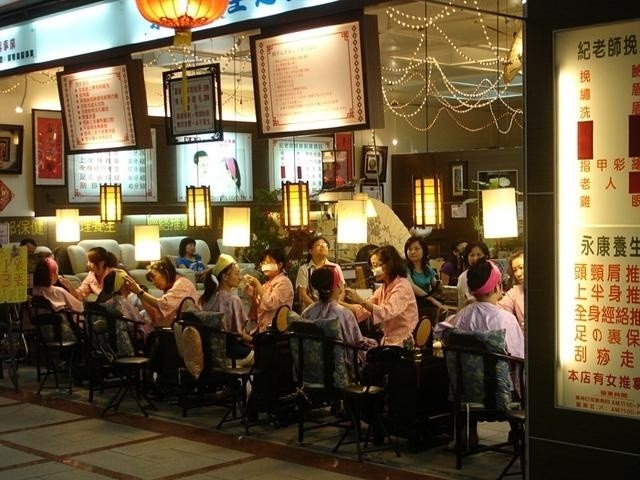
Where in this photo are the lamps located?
[280,136,310,231]
[186,45,211,229]
[337,133,368,243]
[411,0,446,231]
[482,0,519,239]
[135,148,162,262]
[223,35,251,247]
[56,186,80,242]
[98,150,122,224]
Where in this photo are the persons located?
[201,243,295,424]
[99,257,201,392]
[217,158,246,200]
[294,236,445,410]
[174,238,209,283]
[186,150,208,187]
[20,238,37,255]
[440,240,526,443]
[21,247,145,338]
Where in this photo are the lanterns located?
[134,0,229,113]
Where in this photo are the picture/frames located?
[249,17,370,138]
[55,58,138,155]
[162,63,223,145]
[360,182,384,204]
[447,160,468,203]
[32,108,68,188]
[0,124,24,174]
[361,144,388,183]
